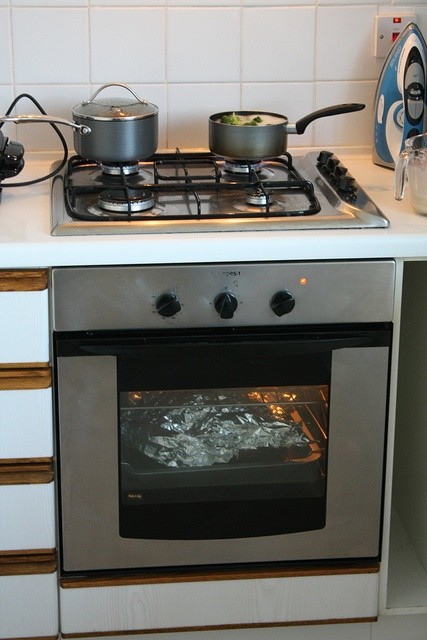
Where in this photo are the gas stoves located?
[49,147,391,237]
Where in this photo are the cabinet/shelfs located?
[0,245,57,639]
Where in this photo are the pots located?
[0,82,160,159]
[208,101,365,161]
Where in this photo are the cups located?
[393,130,427,218]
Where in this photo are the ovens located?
[51,259,397,582]
